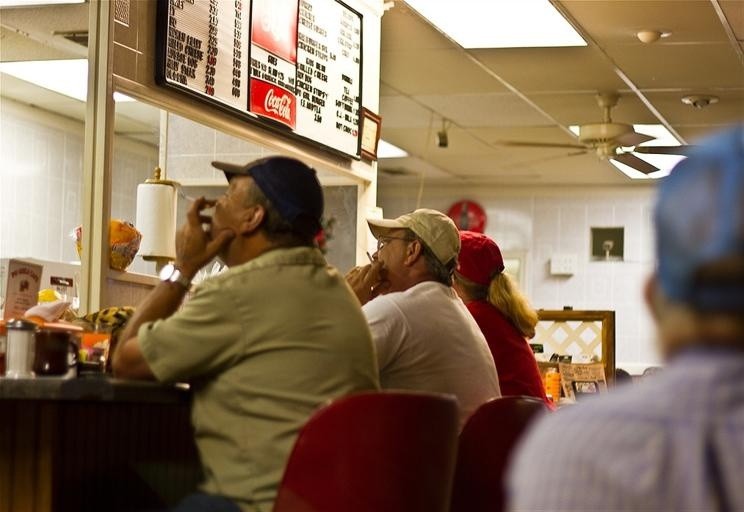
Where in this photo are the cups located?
[33,331,80,378]
[72,321,116,376]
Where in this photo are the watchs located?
[159,263,192,292]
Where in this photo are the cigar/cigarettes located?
[366,251,373,263]
[181,194,212,209]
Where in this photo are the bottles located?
[5,318,37,379]
[541,365,557,400]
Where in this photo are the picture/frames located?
[154,0,364,161]
[501,250,528,293]
[361,107,383,162]
[535,309,616,390]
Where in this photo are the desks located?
[0,374,192,511]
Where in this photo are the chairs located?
[450,394,548,511]
[270,390,462,511]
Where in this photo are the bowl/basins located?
[72,219,143,270]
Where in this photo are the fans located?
[494,91,696,175]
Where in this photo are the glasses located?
[376,234,414,252]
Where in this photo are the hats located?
[649,122,742,318]
[367,207,461,271]
[210,154,325,236]
[456,229,505,286]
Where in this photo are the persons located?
[501,125,743,512]
[111,156,380,512]
[343,208,503,432]
[450,230,554,412]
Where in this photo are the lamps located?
[681,95,719,110]
[137,167,179,263]
